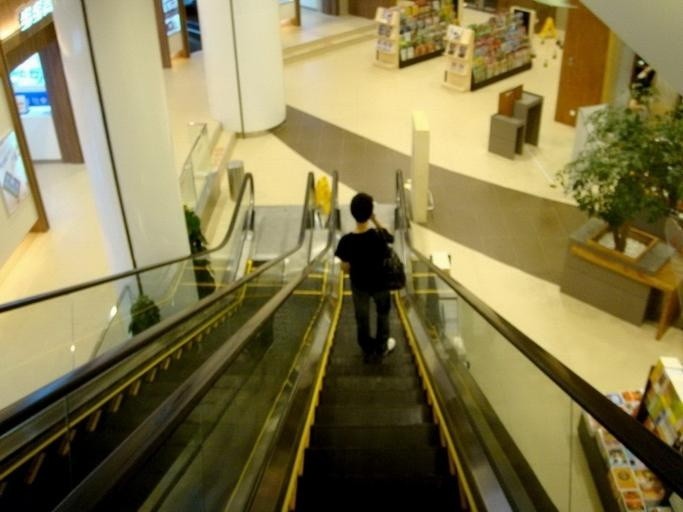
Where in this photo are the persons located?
[334,193,396,357]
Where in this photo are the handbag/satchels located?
[383,248,405,289]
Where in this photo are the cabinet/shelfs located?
[443,12,535,91]
[374,0,456,69]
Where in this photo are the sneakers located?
[383,337,396,357]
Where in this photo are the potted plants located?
[550,81,683,345]
[181,204,218,298]
